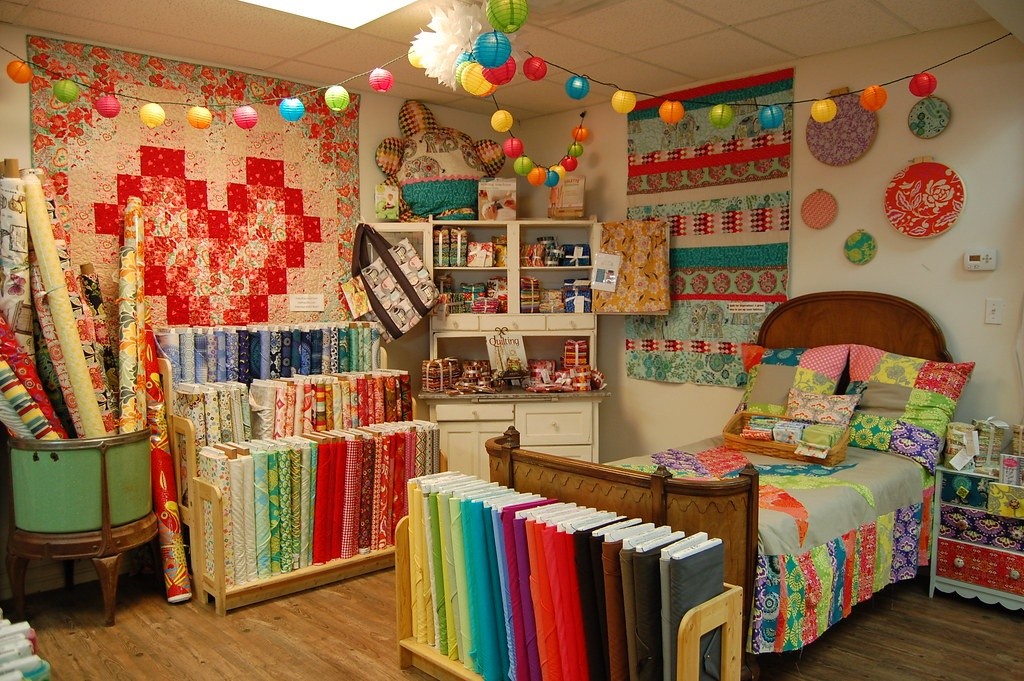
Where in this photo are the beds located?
[485,290,954,680]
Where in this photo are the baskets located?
[723,411,850,466]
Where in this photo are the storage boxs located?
[6,427,153,533]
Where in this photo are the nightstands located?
[929,462,1024,611]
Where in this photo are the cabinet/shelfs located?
[157,346,449,615]
[429,399,598,480]
[429,215,600,331]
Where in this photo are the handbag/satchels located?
[341,223,442,344]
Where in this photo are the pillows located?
[732,343,849,423]
[844,342,975,474]
[785,385,863,424]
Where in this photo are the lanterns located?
[1,0,938,189]
